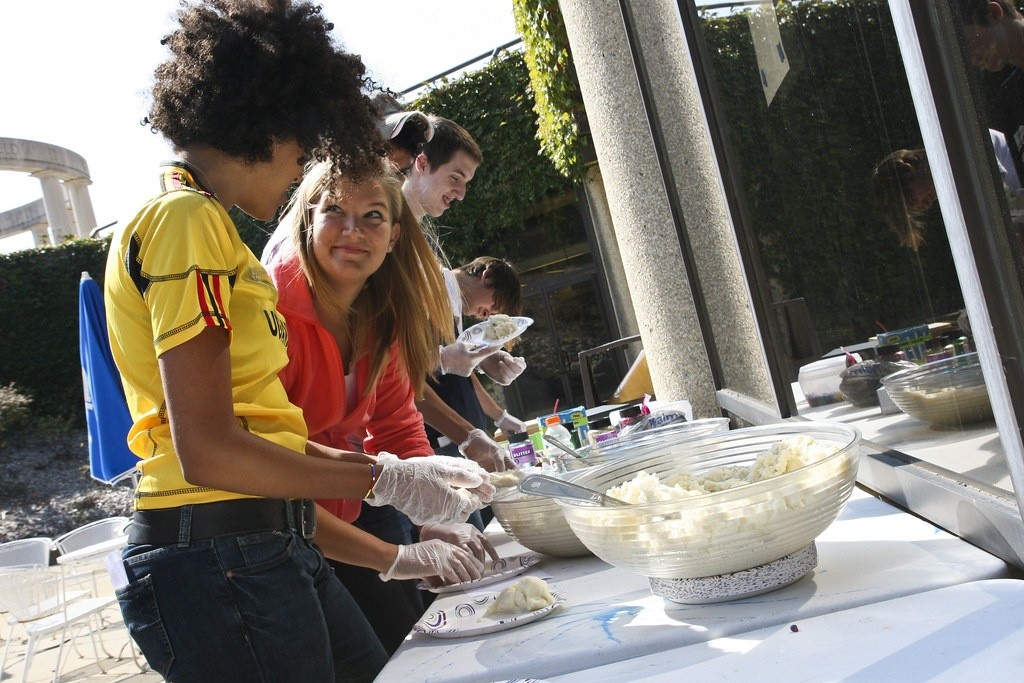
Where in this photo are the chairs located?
[0,515,151,683]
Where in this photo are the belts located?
[127,499,315,544]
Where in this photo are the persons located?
[257,151,500,660]
[451,255,526,438]
[104,0,494,683]
[400,113,519,532]
[874,128,1023,252]
[368,92,502,612]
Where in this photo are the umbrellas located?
[79,271,142,489]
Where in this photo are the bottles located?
[507,432,538,470]
[543,416,576,473]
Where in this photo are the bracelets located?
[363,463,375,500]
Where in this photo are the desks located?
[56,534,130,630]
[790,379,1024,499]
[374,484,1024,683]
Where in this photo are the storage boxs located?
[537,406,591,441]
[610,399,692,422]
[491,425,545,459]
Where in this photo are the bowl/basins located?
[553,421,861,579]
[487,466,599,557]
[879,351,993,425]
[557,417,730,473]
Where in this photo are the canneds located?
[618,405,646,430]
[586,415,620,445]
[507,431,538,468]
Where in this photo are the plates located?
[456,316,534,353]
[416,555,544,593]
[413,590,568,638]
